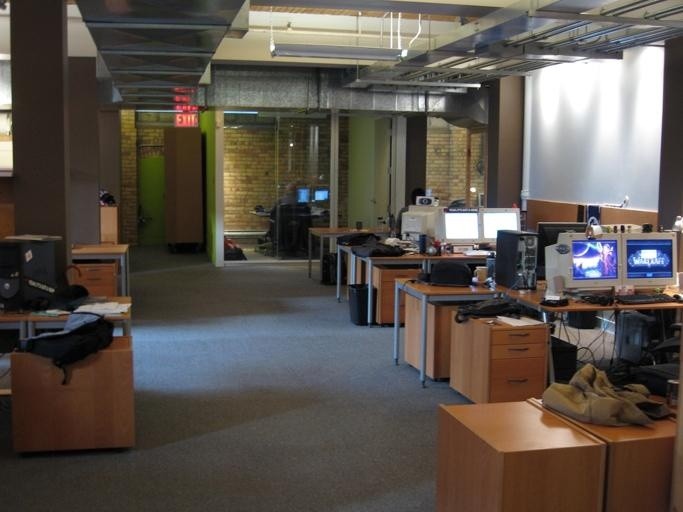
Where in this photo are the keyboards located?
[617,290,671,305]
[462,249,497,257]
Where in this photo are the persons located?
[269,182,312,260]
[394,187,426,241]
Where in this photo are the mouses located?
[669,293,683,304]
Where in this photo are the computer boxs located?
[0,233,66,310]
[494,229,537,291]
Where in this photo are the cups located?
[425,189,432,197]
[474,266,489,282]
[676,272,683,289]
[356,222,363,230]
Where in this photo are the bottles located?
[435,197,439,206]
[377,216,386,233]
[434,240,441,256]
[419,234,426,254]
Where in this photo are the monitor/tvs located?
[543,232,621,297]
[312,184,330,202]
[623,234,677,294]
[479,206,522,244]
[401,204,442,244]
[441,208,481,251]
[536,219,588,277]
[296,184,312,204]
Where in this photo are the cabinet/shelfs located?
[71,258,118,296]
[10,337,137,453]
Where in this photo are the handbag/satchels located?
[336,232,420,258]
[321,253,347,285]
[430,261,472,287]
[455,297,522,323]
[17,312,114,369]
[541,362,674,427]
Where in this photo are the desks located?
[1,295,131,340]
[70,243,131,298]
[437,398,677,511]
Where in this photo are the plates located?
[472,276,493,286]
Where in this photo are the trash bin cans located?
[348,283,377,326]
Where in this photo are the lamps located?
[270,8,425,62]
